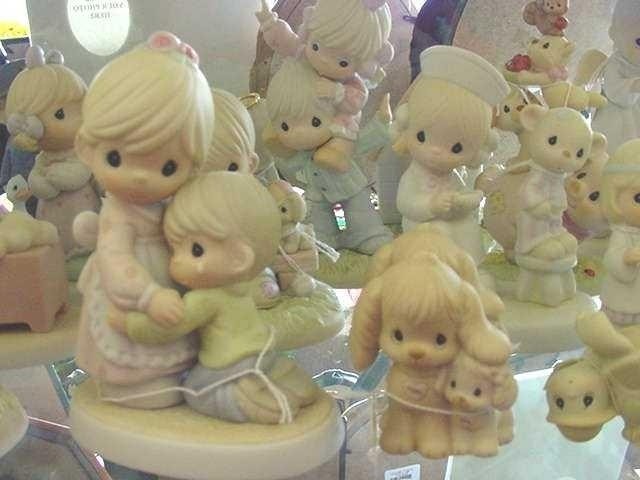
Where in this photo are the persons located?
[0,0,640,459]
[604,139,639,322]
[9,46,98,259]
[251,1,508,318]
[79,27,328,424]
